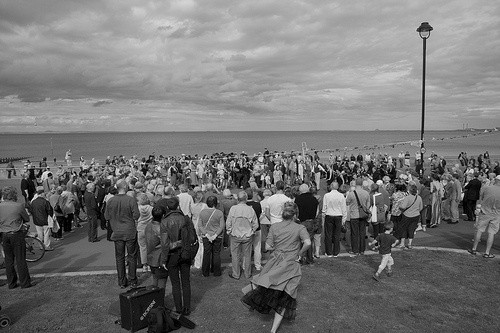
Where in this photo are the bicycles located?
[0,224,45,269]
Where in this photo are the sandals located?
[483,251,495,257]
[468,248,477,254]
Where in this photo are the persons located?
[190,191,207,269]
[322,181,346,258]
[399,185,424,248]
[294,183,320,265]
[468,167,500,258]
[368,220,398,281]
[6,162,16,179]
[240,202,311,333]
[104,179,140,289]
[21,147,500,258]
[226,191,259,279]
[266,180,292,225]
[135,193,153,272]
[0,187,34,290]
[145,197,199,316]
[199,195,225,277]
[346,179,370,255]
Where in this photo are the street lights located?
[416,22,433,164]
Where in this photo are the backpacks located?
[148,305,181,333]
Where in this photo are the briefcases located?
[119,285,165,333]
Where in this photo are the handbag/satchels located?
[195,242,204,269]
[368,194,377,222]
[359,204,372,218]
